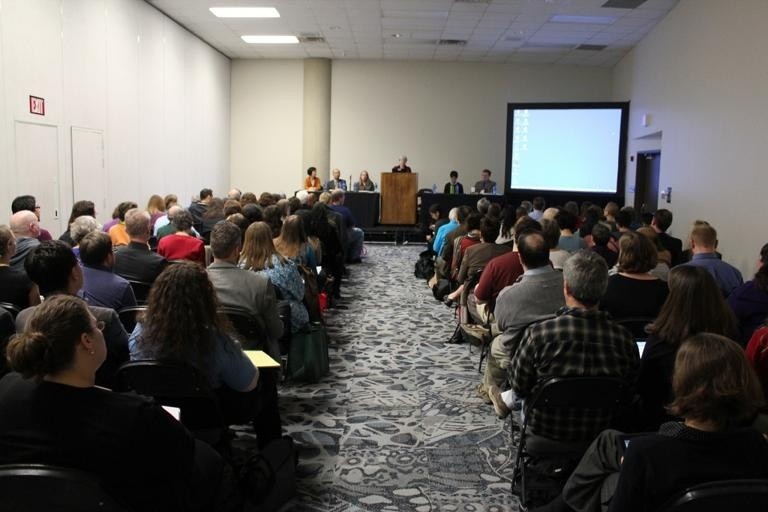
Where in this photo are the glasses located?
[77,322,105,345]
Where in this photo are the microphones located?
[349,176,352,192]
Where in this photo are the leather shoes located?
[325,276,334,289]
[442,294,453,306]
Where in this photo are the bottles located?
[373,182,377,192]
[450,185,454,195]
[341,181,346,189]
[455,184,459,194]
[432,184,437,193]
[492,186,496,194]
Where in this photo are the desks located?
[292,189,382,229]
[420,191,625,231]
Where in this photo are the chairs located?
[449,254,766,512]
[0,204,345,512]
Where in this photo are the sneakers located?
[489,385,512,418]
[476,384,493,405]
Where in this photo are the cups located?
[323,184,327,192]
[471,187,475,192]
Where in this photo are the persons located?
[391,154,412,173]
[443,171,465,193]
[430,197,768,512]
[472,168,500,193]
[0,188,364,512]
[327,169,347,192]
[352,170,374,190]
[303,167,322,191]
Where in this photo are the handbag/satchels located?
[433,279,452,300]
[448,323,491,346]
[282,319,329,384]
[415,249,438,278]
